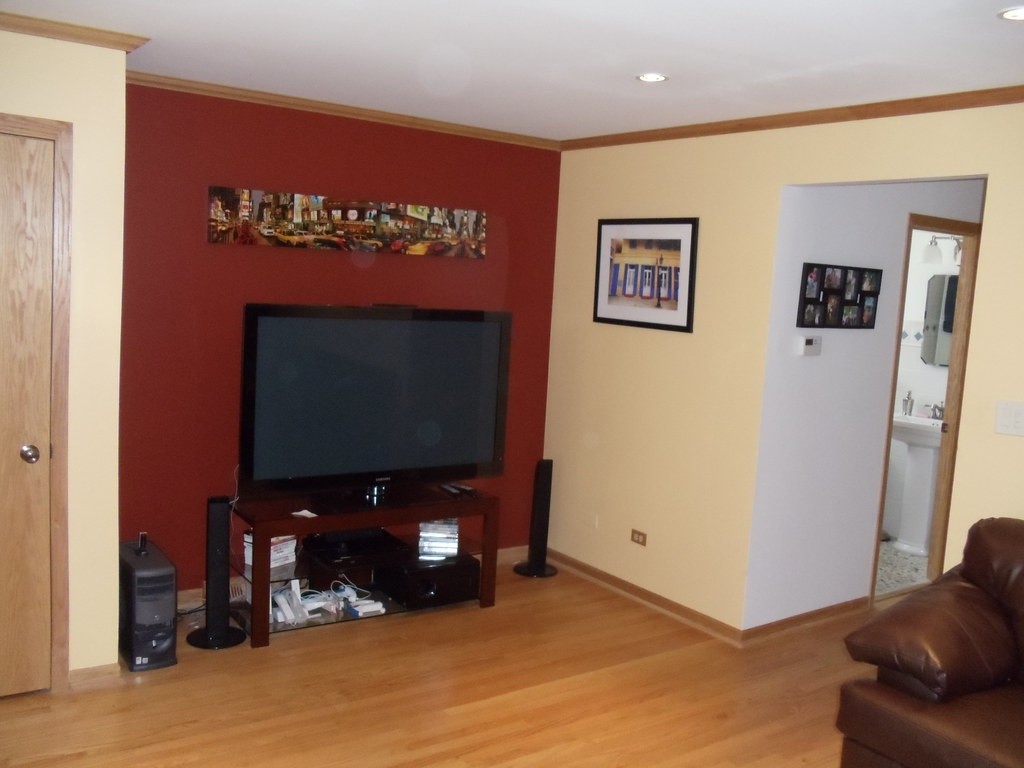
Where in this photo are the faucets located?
[929,403,944,419]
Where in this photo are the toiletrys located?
[900,390,915,415]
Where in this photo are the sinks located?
[891,413,944,448]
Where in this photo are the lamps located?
[920,232,964,267]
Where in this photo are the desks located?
[227,479,503,648]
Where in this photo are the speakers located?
[513,459,557,577]
[186,496,246,649]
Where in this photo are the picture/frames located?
[591,217,698,334]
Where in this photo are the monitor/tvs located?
[237,302,512,493]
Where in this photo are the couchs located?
[835,517,1024,768]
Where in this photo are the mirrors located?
[920,272,959,367]
